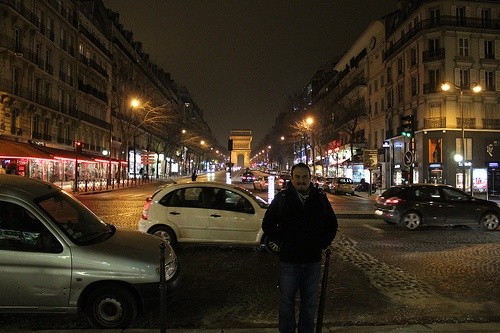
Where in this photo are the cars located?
[374,182,500,231]
[137,181,281,257]
[0,172,184,330]
[232,170,356,196]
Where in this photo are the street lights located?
[304,116,315,166]
[182,129,186,173]
[440,80,482,192]
[128,97,142,186]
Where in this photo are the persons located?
[215,189,228,202]
[261,162,339,333]
[192,170,197,181]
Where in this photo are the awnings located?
[0,140,126,164]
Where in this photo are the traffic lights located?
[401,114,414,138]
